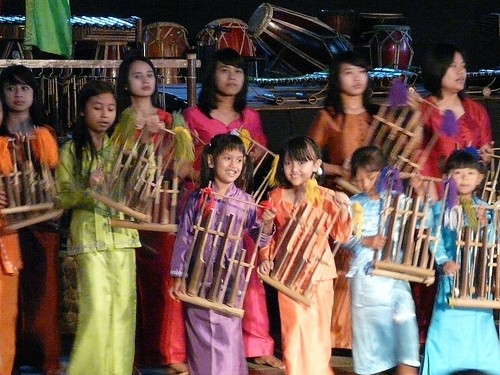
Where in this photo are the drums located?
[359,12,405,61]
[197,18,257,77]
[247,3,357,76]
[318,9,357,43]
[367,24,414,71]
[141,22,190,85]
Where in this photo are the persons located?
[310,51,423,349]
[175,48,285,369]
[169,133,278,374]
[339,145,439,375]
[412,47,494,200]
[107,55,189,374]
[418,146,500,375]
[0,65,59,375]
[257,136,353,374]
[54,80,165,374]
